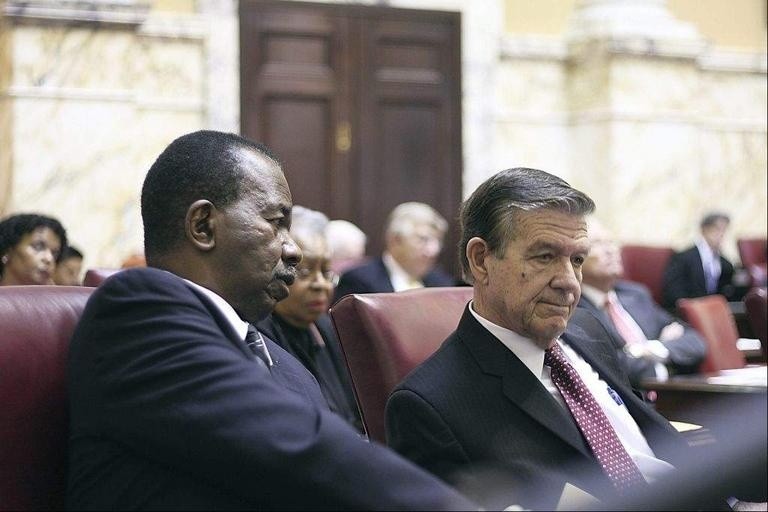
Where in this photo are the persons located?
[330,199,455,299]
[567,208,706,386]
[251,203,365,438]
[61,129,530,512]
[324,217,367,275]
[383,166,766,512]
[660,212,750,309]
[51,246,83,287]
[0,211,66,287]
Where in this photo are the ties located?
[545,344,650,501]
[244,323,270,369]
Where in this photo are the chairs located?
[0,239,768,512]
